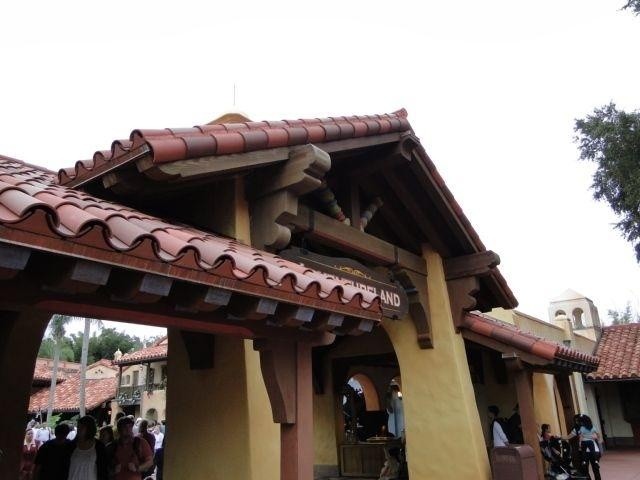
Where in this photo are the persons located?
[378,374,408,480]
[541,413,602,480]
[19,397,165,480]
[487,401,523,447]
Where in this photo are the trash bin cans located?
[493,444,539,480]
